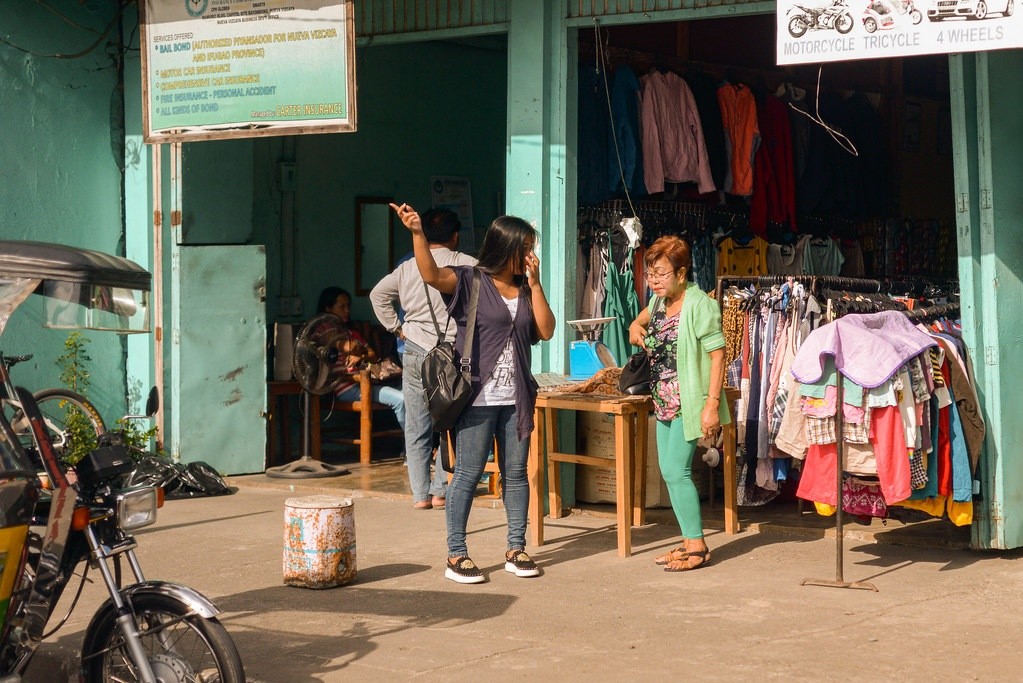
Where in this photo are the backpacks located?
[421,266,481,432]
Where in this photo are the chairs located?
[296,321,404,465]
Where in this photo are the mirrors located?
[355,197,394,298]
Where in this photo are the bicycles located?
[0,350,108,493]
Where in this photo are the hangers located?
[723,67,743,92]
[719,275,962,326]
[577,200,860,257]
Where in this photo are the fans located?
[265,314,352,479]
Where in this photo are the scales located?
[567,317,618,381]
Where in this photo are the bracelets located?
[707,394,722,403]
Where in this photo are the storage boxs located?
[578,411,711,508]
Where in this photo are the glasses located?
[641,267,679,282]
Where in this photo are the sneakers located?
[504,550,539,576]
[444,556,484,583]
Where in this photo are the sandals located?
[664,546,712,571]
[657,548,686,564]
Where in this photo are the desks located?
[526,392,654,557]
[265,382,301,467]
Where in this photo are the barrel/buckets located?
[283,495,357,588]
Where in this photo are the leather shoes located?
[416,498,434,511]
[433,498,445,510]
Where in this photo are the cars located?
[926,0,1016,22]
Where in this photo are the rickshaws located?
[0,236,250,683]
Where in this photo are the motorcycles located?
[860,0,923,34]
[785,0,855,39]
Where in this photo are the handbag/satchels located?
[620,353,650,394]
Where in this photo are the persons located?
[629,234,732,572]
[388,202,556,585]
[369,208,479,509]
[308,286,405,432]
